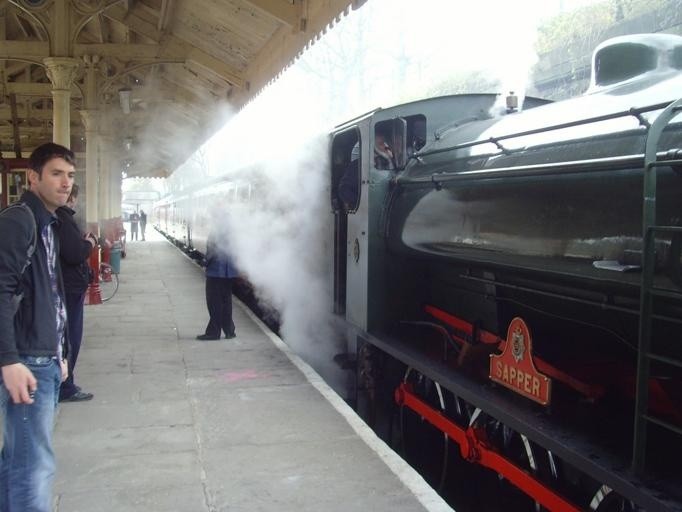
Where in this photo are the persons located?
[338,133,395,211]
[54,181,99,402]
[139,209,148,242]
[197,196,246,341]
[129,210,138,238]
[2,139,73,511]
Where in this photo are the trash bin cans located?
[110,241,122,274]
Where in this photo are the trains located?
[147,29,682,512]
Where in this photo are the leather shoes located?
[224,331,237,339]
[197,332,219,341]
[61,390,93,402]
